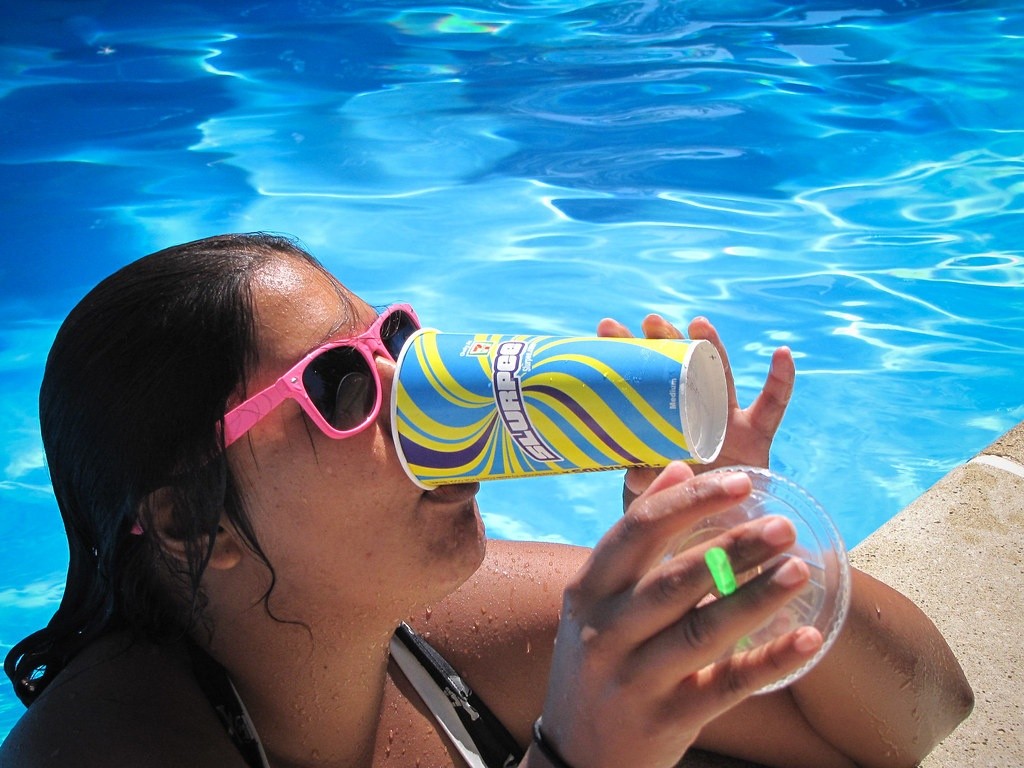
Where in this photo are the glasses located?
[128,302,423,536]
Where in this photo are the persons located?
[0,233,975,768]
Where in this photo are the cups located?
[391,327,728,491]
